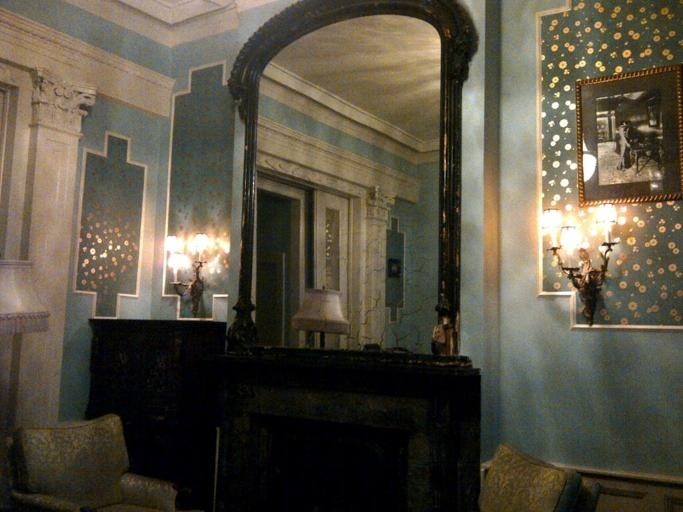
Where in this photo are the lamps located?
[164,232,230,317]
[289,285,352,351]
[0,258,50,431]
[540,202,620,326]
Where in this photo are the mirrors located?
[226,1,479,357]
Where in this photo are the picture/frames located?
[572,63,682,208]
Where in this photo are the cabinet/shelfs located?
[83,319,226,509]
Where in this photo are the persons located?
[615,119,635,173]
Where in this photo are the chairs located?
[0,414,179,511]
[476,439,601,511]
